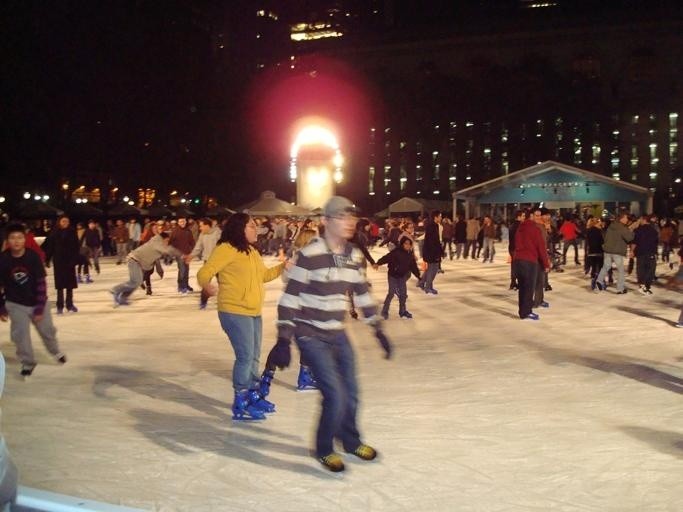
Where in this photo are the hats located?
[323,198,359,220]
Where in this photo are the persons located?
[1,223,71,383]
[354,211,505,320]
[198,213,287,420]
[266,197,389,470]
[0,216,324,314]
[509,206,682,319]
[260,238,320,395]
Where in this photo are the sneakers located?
[320,453,344,473]
[415,282,438,294]
[55,352,66,364]
[66,305,77,312]
[541,302,549,307]
[520,312,538,320]
[400,311,412,318]
[353,442,376,460]
[20,364,35,376]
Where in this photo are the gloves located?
[266,338,292,370]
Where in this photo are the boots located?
[297,365,318,386]
[232,369,274,416]
[77,276,94,283]
